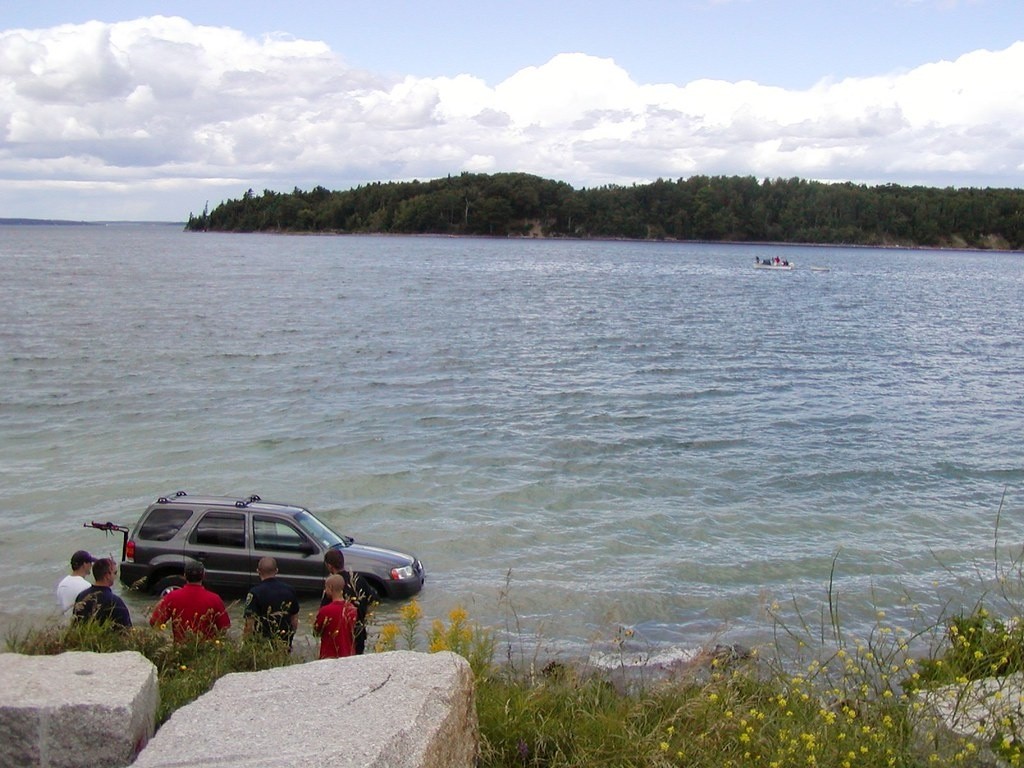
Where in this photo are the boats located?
[752,261,794,271]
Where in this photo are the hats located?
[71,550,98,563]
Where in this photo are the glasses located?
[105,571,118,574]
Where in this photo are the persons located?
[320,549,381,655]
[72,558,133,628]
[753,255,790,267]
[312,575,359,660]
[149,561,233,648]
[59,550,97,619]
[244,557,299,654]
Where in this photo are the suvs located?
[119,489,426,607]
